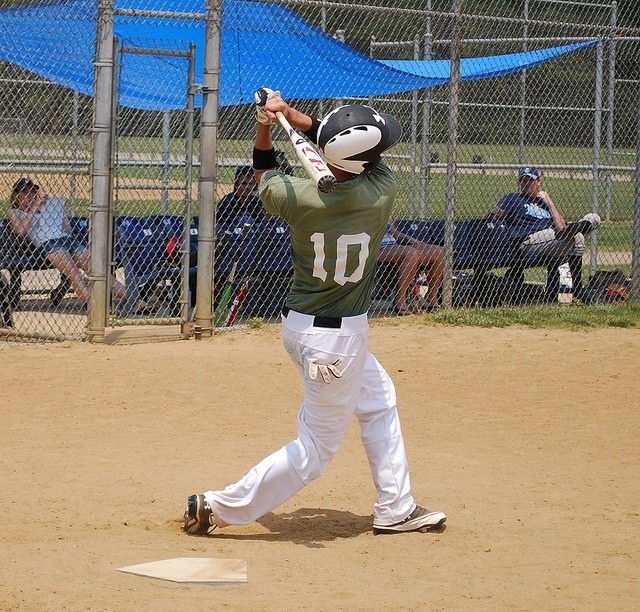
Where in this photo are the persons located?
[486,167,602,306]
[182,84,447,535]
[6,177,159,316]
[217,165,267,217]
[376,214,446,316]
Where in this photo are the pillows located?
[317,105,402,175]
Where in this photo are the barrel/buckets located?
[421,305,442,312]
[393,300,412,316]
[132,305,159,315]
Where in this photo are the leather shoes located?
[254,87,273,127]
[309,356,342,384]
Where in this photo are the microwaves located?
[214,262,238,327]
[223,277,249,328]
[274,110,339,194]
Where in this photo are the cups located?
[235,181,257,186]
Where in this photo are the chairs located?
[572,293,595,305]
[373,505,446,532]
[554,222,585,241]
[0,213,563,314]
[184,494,217,536]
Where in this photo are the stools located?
[281,306,341,329]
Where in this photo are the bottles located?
[12,178,39,192]
[519,167,539,179]
